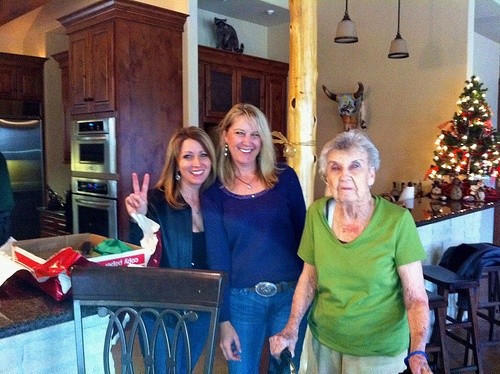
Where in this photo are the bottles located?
[391,181,424,202]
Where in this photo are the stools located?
[423,264,500,374]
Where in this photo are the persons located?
[0,151,15,238]
[200,102,307,372]
[125,124,217,374]
[268,129,432,374]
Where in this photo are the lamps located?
[334,0,358,44]
[387,0,410,60]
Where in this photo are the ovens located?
[69,119,118,240]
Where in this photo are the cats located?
[214,16,245,54]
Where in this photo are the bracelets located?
[404,350,430,366]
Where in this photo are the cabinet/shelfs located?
[0,0,191,239]
[197,45,290,163]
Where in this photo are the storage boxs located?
[8,231,147,301]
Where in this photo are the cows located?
[322,80,368,133]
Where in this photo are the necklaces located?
[188,194,200,215]
[235,175,257,188]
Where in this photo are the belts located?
[244,279,299,298]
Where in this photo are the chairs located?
[68,265,226,374]
